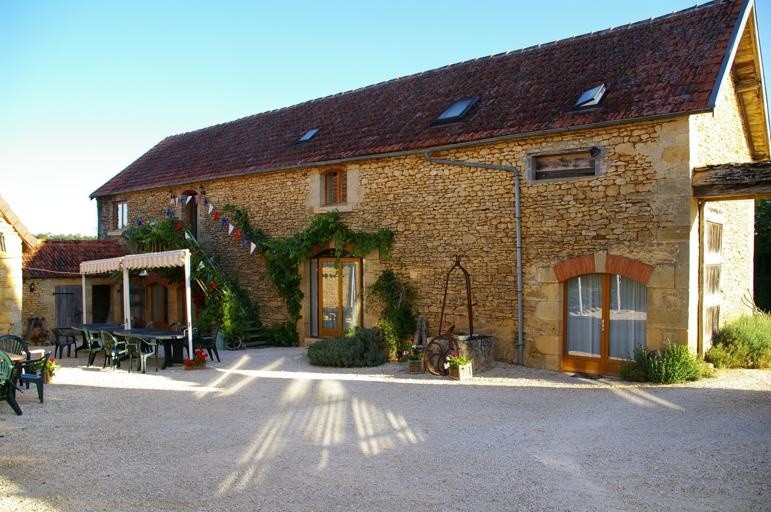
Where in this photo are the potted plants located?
[407,352,426,374]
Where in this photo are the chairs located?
[0,334,53,416]
[51,320,221,373]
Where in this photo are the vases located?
[447,363,473,380]
[43,368,50,383]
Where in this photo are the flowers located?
[31,356,57,373]
[443,352,469,370]
[29,329,49,346]
[183,347,208,368]
